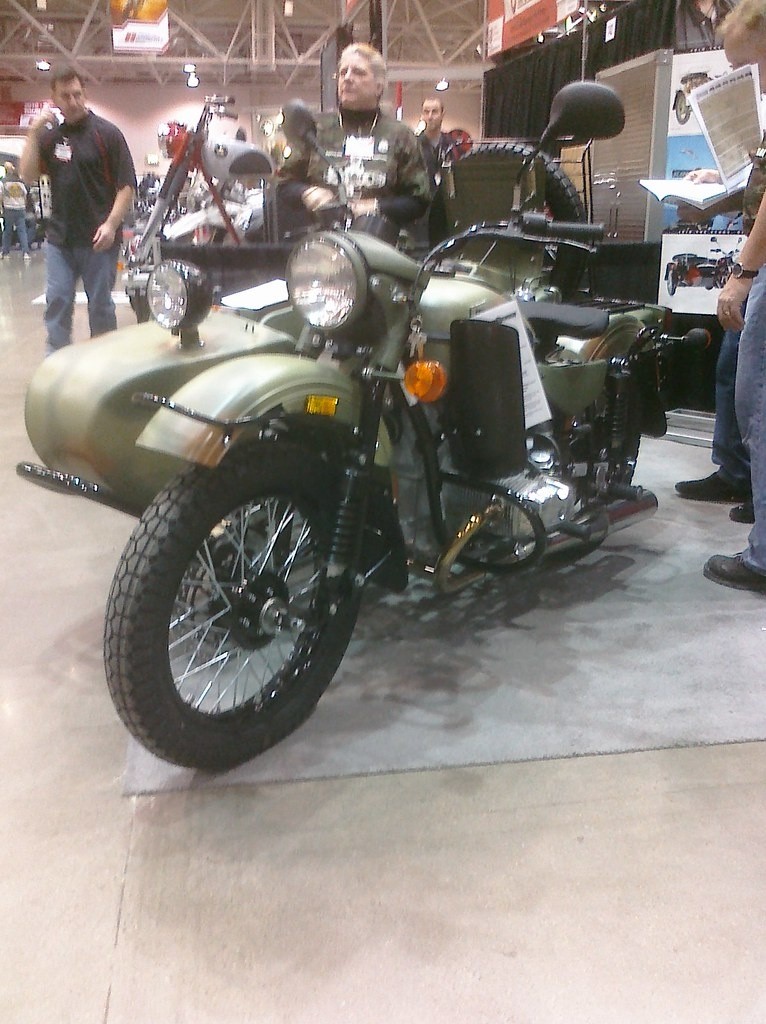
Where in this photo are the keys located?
[408,326,427,358]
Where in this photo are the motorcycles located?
[119,95,444,321]
[671,71,728,125]
[15,76,716,775]
[664,236,744,296]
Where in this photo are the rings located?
[723,311,730,315]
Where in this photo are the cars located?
[0,149,46,253]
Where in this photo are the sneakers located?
[702,554,765,592]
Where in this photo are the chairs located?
[520,300,610,354]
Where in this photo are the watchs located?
[732,263,759,278]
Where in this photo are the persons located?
[675,0,766,591]
[417,98,460,189]
[17,65,135,358]
[277,42,438,220]
[0,162,30,259]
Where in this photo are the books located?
[640,179,747,210]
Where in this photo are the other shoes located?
[728,504,755,525]
[23,253,31,260]
[2,253,10,259]
[674,472,751,503]
